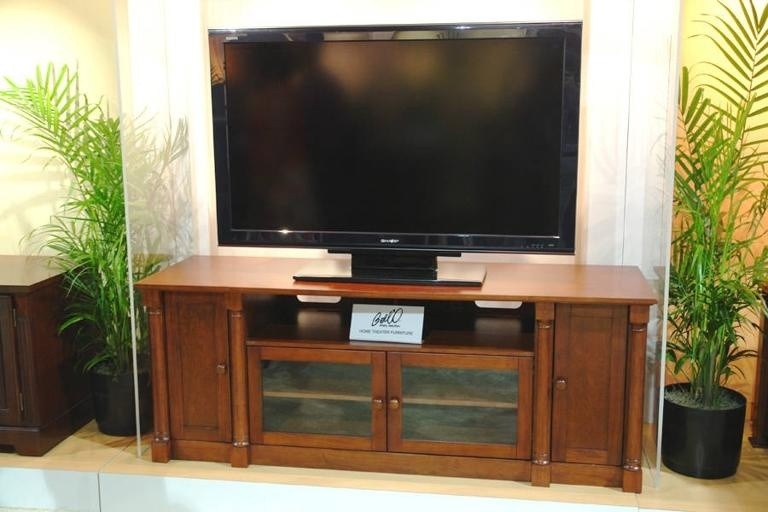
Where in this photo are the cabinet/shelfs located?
[0,254,101,458]
[134,255,658,494]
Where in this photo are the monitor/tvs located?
[208,21,582,287]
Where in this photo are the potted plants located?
[662,0,768,480]
[0,62,193,438]
